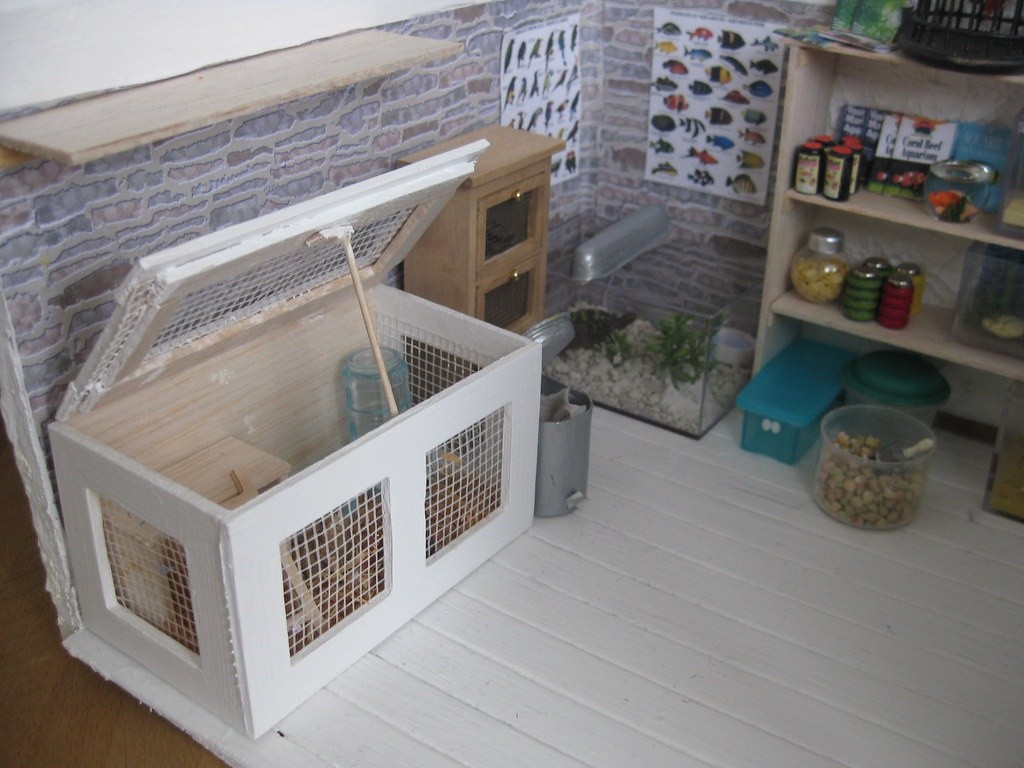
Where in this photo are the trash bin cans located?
[522,310,594,519]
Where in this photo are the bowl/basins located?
[811,403,937,531]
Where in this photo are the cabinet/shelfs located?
[749,27,1024,519]
[394,124,569,415]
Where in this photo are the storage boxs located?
[539,170,770,440]
[46,139,544,739]
[736,340,854,467]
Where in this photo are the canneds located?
[795,136,862,201]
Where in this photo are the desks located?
[103,439,292,630]
